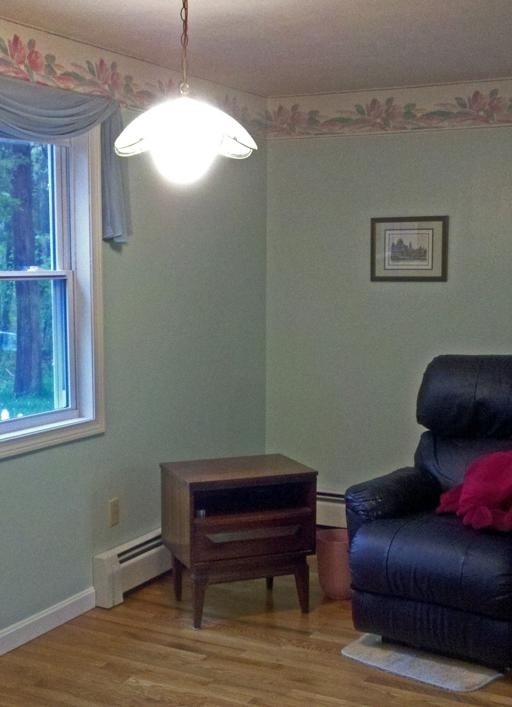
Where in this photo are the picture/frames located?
[369,215,451,284]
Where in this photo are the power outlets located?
[108,497,120,526]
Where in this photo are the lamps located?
[113,1,259,188]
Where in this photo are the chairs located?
[344,354,512,677]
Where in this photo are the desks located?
[160,453,318,628]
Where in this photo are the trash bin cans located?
[317,529,349,598]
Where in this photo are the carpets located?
[342,634,504,695]
